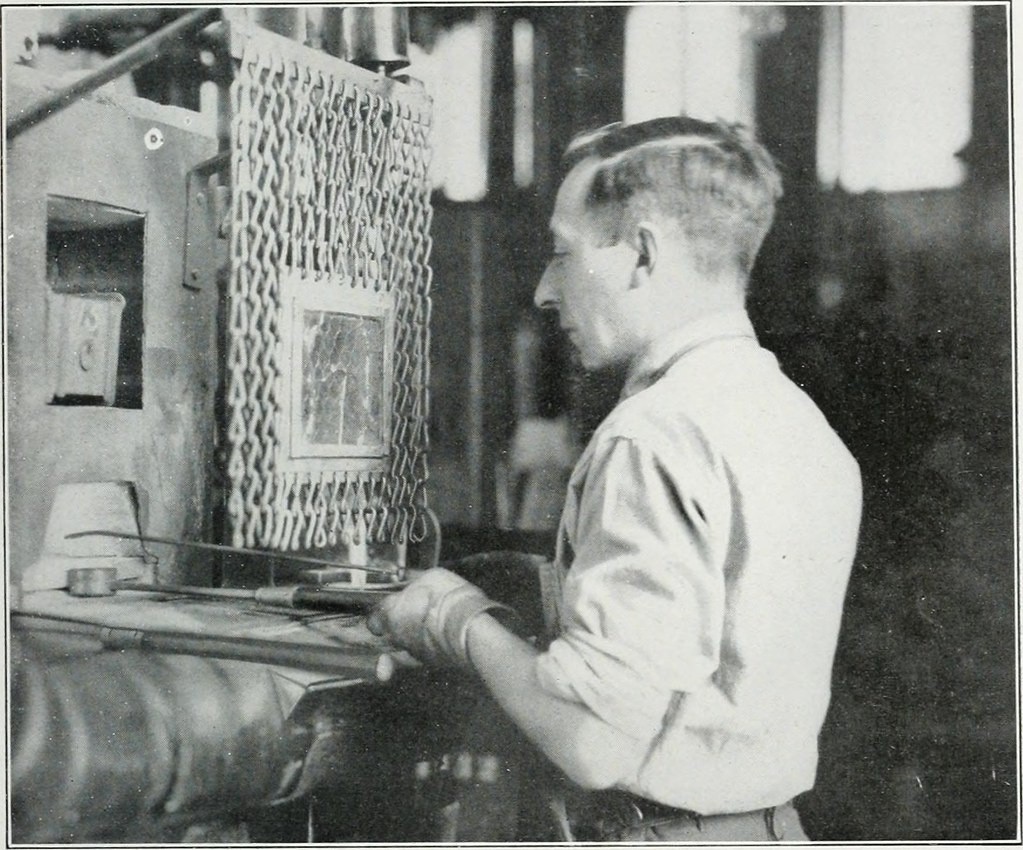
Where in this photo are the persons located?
[366,117,864,842]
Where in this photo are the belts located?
[597,801,690,829]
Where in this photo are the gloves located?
[368,564,528,679]
